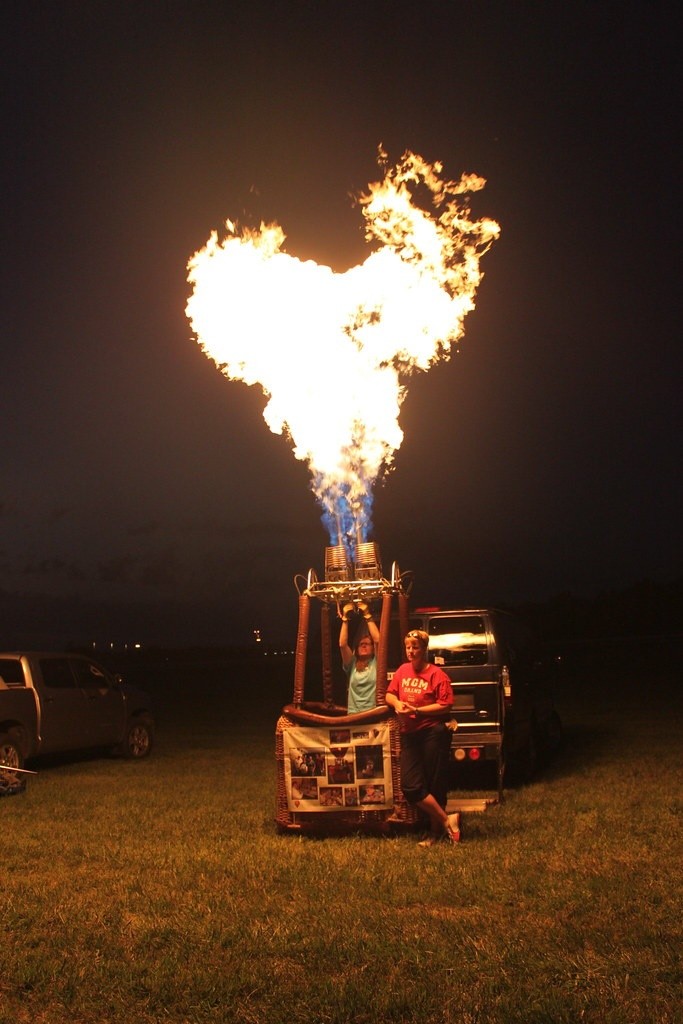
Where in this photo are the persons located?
[384,628,462,850]
[341,601,382,718]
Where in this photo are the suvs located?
[354,610,562,785]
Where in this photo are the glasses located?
[405,633,426,645]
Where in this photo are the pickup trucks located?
[0,651,155,774]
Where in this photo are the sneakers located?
[418,831,441,848]
[445,812,461,846]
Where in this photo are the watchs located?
[414,707,419,717]
